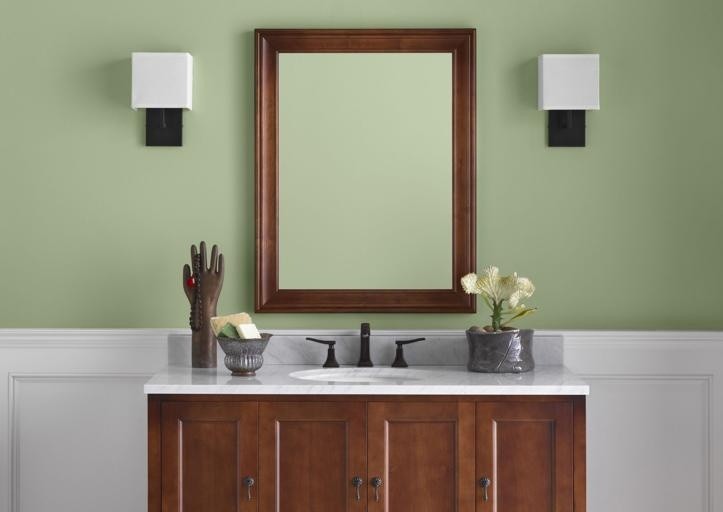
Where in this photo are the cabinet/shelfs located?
[146,395,260,511]
[477,398,574,511]
[259,395,475,512]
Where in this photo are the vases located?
[466,324,536,373]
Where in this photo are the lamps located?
[130,52,194,148]
[537,53,601,147]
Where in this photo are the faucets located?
[357,323,374,367]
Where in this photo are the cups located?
[215,332,271,377]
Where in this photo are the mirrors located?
[253,26,477,314]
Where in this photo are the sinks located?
[288,367,427,383]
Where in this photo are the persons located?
[182,242,224,368]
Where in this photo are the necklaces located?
[186,254,203,332]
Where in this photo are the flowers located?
[461,268,539,331]
